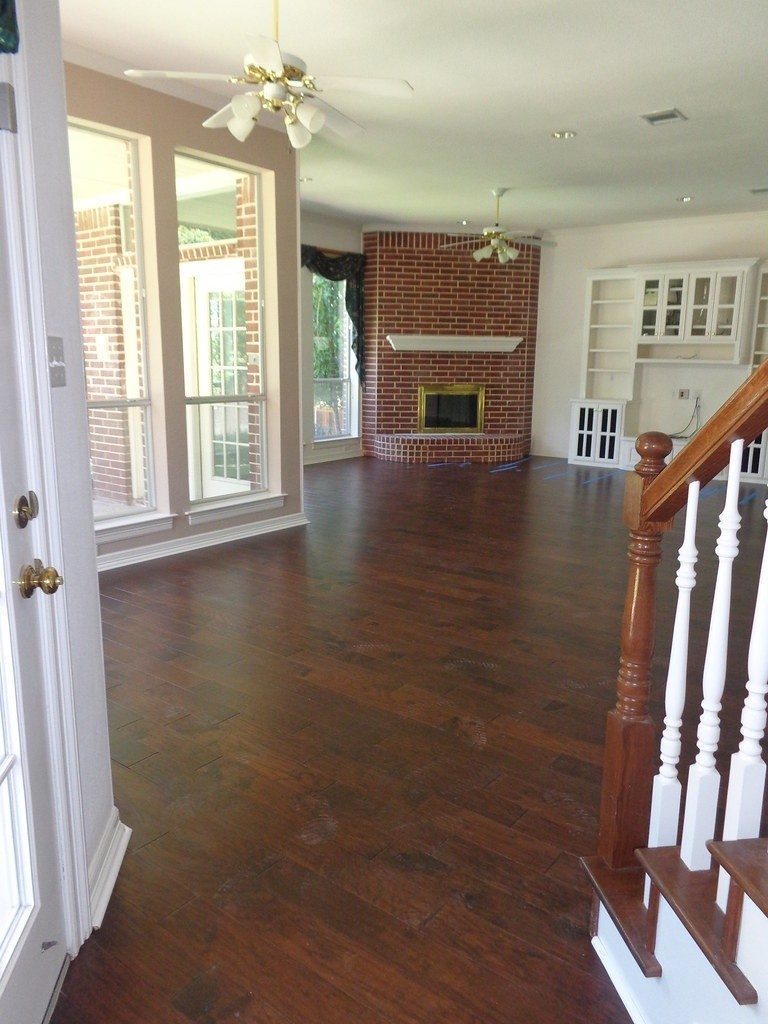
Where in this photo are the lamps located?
[202,51,327,150]
[473,227,520,263]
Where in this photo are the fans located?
[123,0,414,139]
[440,188,557,248]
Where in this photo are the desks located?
[313,404,344,434]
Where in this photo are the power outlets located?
[679,389,689,399]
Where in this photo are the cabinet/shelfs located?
[568,256,768,484]
